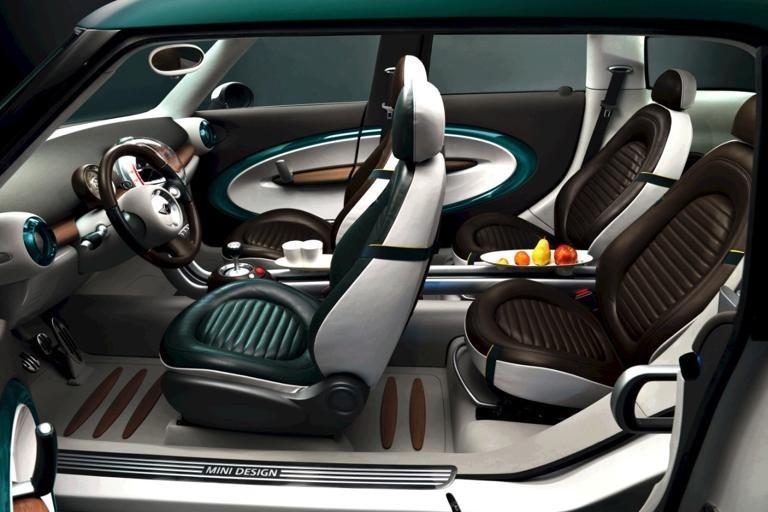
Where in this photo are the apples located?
[554,246,577,265]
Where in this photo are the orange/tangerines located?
[515,252,530,266]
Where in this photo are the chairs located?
[457,67,697,265]
[469,93,755,405]
[153,77,455,434]
[221,56,432,261]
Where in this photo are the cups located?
[282,239,300,264]
[300,239,323,265]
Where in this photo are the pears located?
[532,236,551,266]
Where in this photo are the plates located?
[478,248,592,272]
